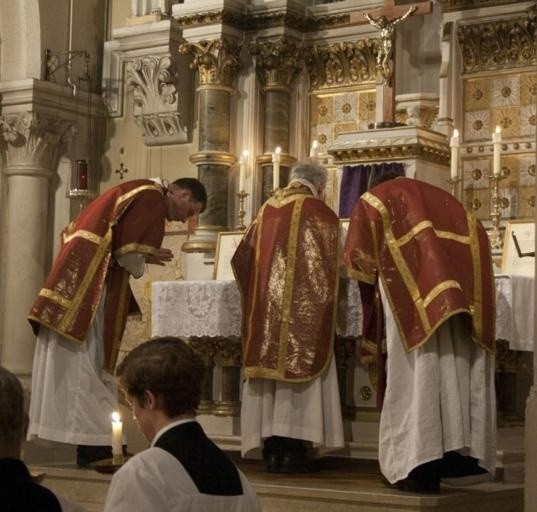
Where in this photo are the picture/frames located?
[211,231,248,282]
[339,217,352,254]
[499,219,537,274]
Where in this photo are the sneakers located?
[76,446,113,466]
[266,454,321,473]
[376,464,442,495]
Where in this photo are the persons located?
[101,333,262,512]
[340,174,502,495]
[229,161,364,476]
[0,363,69,512]
[24,177,207,468]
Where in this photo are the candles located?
[238,140,321,195]
[110,409,123,459]
[449,125,502,180]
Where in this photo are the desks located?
[143,273,533,419]
